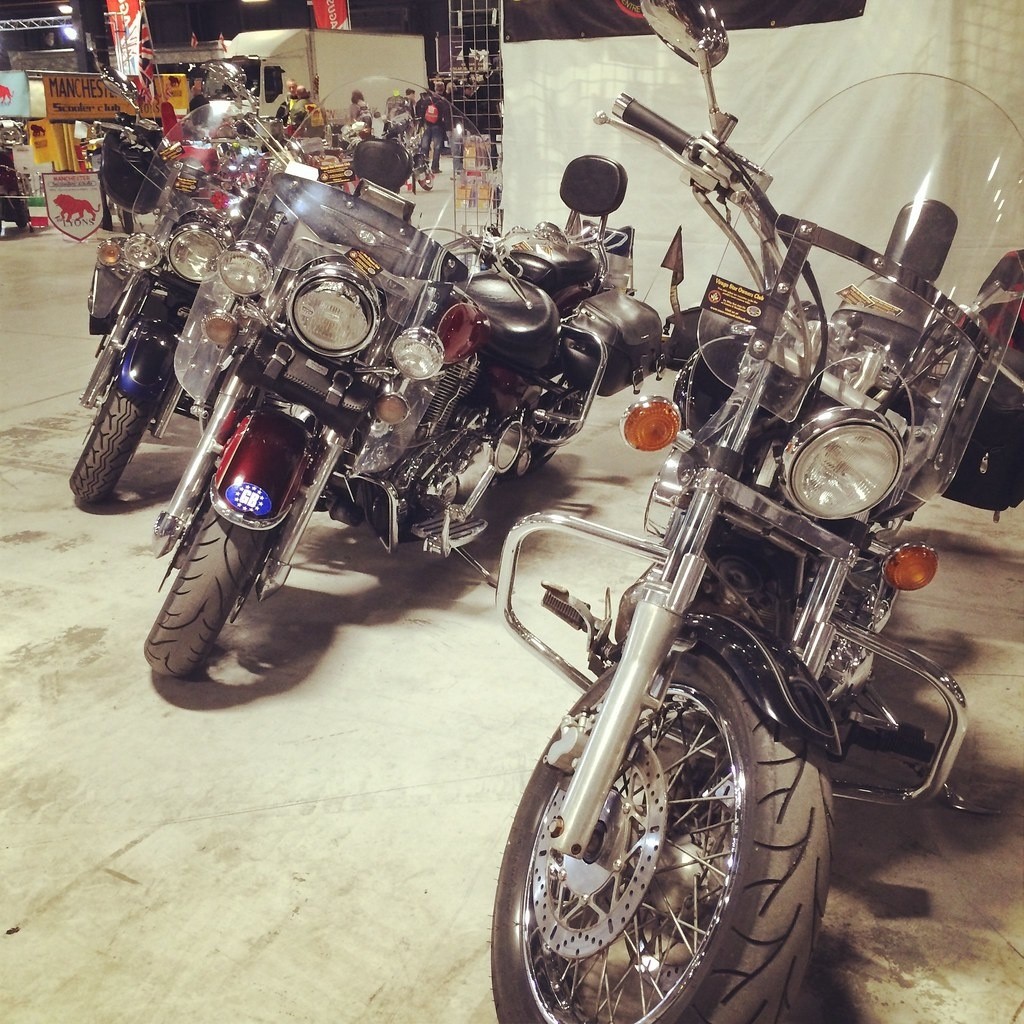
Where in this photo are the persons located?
[350,56,503,174]
[276,78,326,138]
[188,86,209,127]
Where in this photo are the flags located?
[137,1,156,104]
[219,33,227,51]
[190,32,198,49]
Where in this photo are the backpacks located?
[425,97,441,123]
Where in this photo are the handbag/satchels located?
[938,249,1024,510]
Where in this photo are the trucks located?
[0,55,291,236]
[219,29,430,150]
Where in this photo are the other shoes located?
[432,169,441,173]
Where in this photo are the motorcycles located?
[487,0,1024,1024]
[65,58,664,681]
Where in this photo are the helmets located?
[99,132,166,215]
